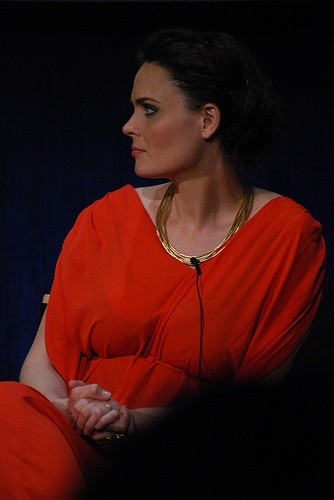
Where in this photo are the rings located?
[107,434,123,440]
[105,404,113,410]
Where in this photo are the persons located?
[0,18,326,500]
[0,352,334,500]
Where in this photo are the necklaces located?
[155,182,254,266]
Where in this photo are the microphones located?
[191,257,201,274]
